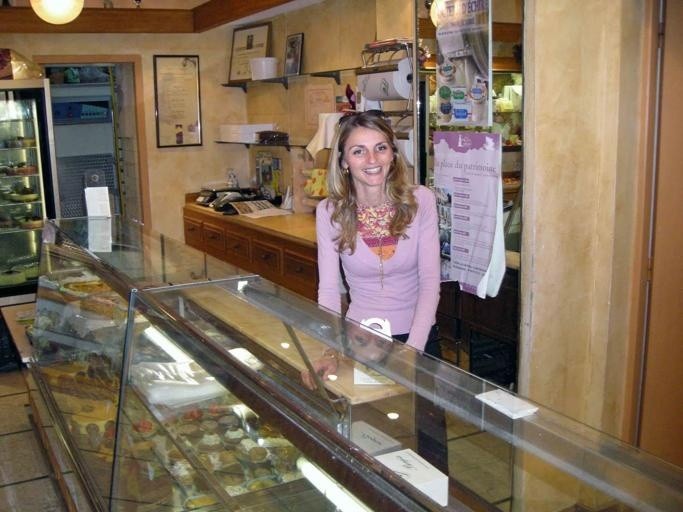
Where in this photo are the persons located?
[300,110,450,475]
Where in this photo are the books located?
[229,199,293,220]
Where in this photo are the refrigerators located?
[0,78,63,307]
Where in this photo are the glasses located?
[338,110,385,127]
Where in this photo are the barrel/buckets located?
[249,57,280,81]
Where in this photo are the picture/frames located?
[151,54,202,148]
[228,21,303,83]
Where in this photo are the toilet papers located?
[356,56,414,101]
[306,112,342,160]
[395,126,414,168]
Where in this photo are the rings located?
[324,350,338,361]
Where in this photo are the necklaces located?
[352,196,395,289]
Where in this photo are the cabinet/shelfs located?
[182,193,317,301]
[212,77,291,152]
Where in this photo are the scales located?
[196,188,256,206]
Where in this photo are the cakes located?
[41,371,296,512]
